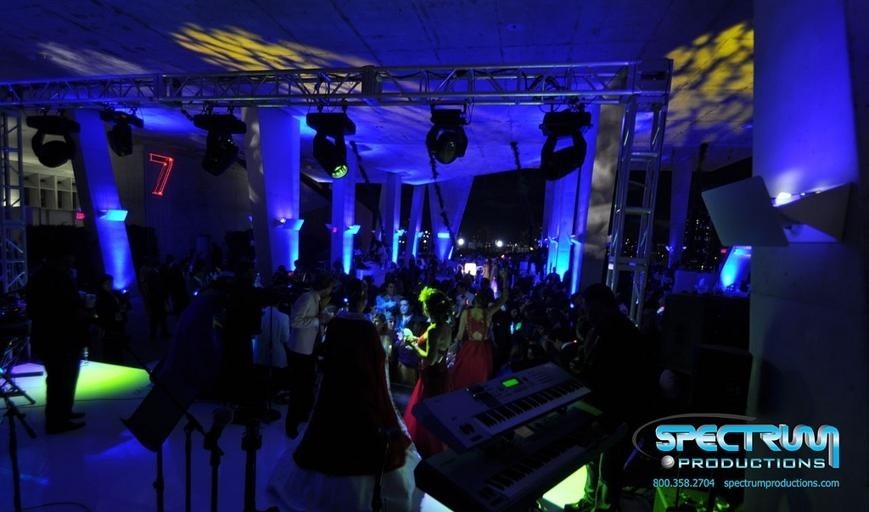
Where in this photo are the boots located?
[371,486,381,512]
[203,407,232,456]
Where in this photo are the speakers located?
[681,346,753,425]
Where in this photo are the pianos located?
[414,401,610,512]
[412,362,591,454]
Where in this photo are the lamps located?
[537,92,592,180]
[99,108,145,158]
[191,113,248,177]
[26,114,80,168]
[426,100,470,164]
[306,111,356,179]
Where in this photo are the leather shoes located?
[285,414,298,438]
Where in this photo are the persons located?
[31,222,698,512]
